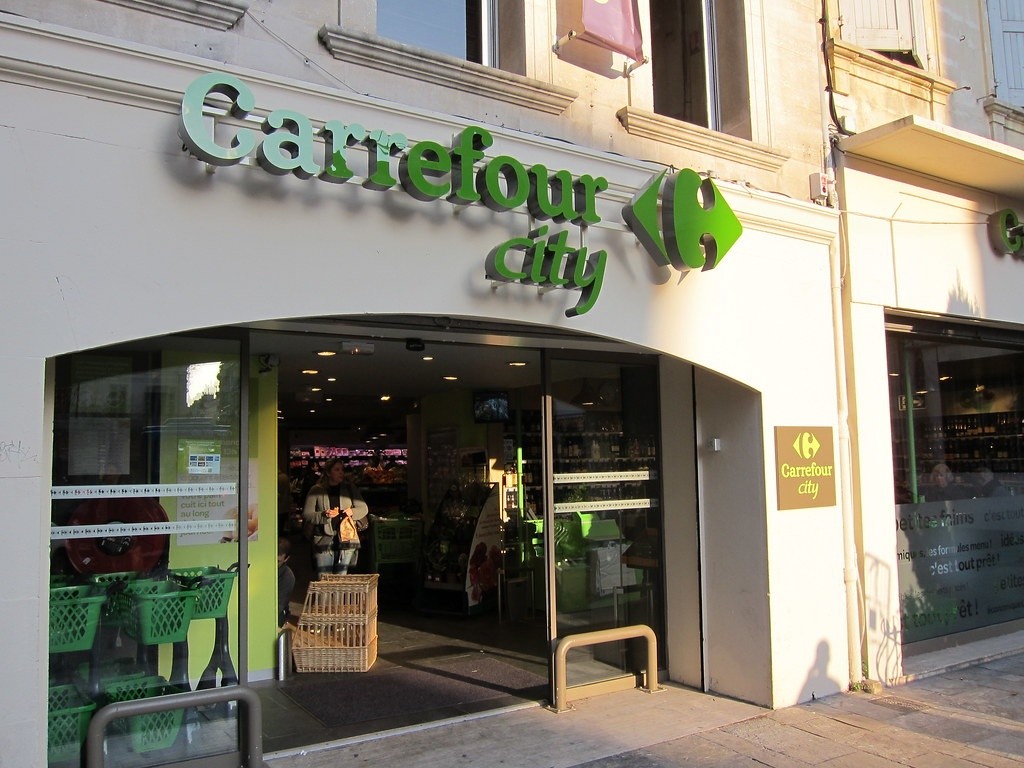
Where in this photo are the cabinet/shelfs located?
[290,454,411,493]
[893,411,1022,505]
[520,429,660,519]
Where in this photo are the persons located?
[278,468,292,538]
[301,458,368,604]
[924,463,967,502]
[277,537,296,616]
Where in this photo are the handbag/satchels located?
[356,516,369,532]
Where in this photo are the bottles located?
[915,414,1024,481]
[524,420,661,516]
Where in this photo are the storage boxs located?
[47,566,238,765]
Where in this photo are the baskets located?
[49,566,236,653]
[291,573,380,673]
[49,663,187,764]
